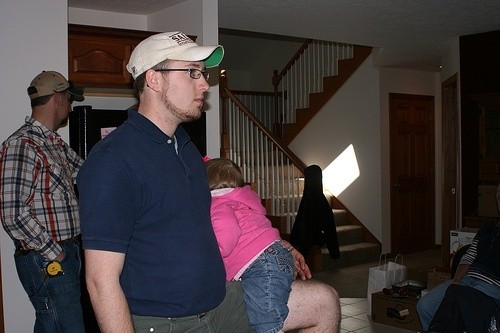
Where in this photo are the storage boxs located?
[449,228,478,265]
[372,288,423,332]
[428,265,451,289]
[392,279,426,297]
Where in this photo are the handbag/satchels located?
[367,252,408,316]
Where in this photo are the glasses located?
[147,68,210,87]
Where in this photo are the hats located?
[28,70,85,102]
[125,31,225,81]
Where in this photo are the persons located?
[427,180,500,333]
[0,71,85,333]
[450,241,462,267]
[102,128,110,136]
[206,158,295,333]
[77,31,341,333]
